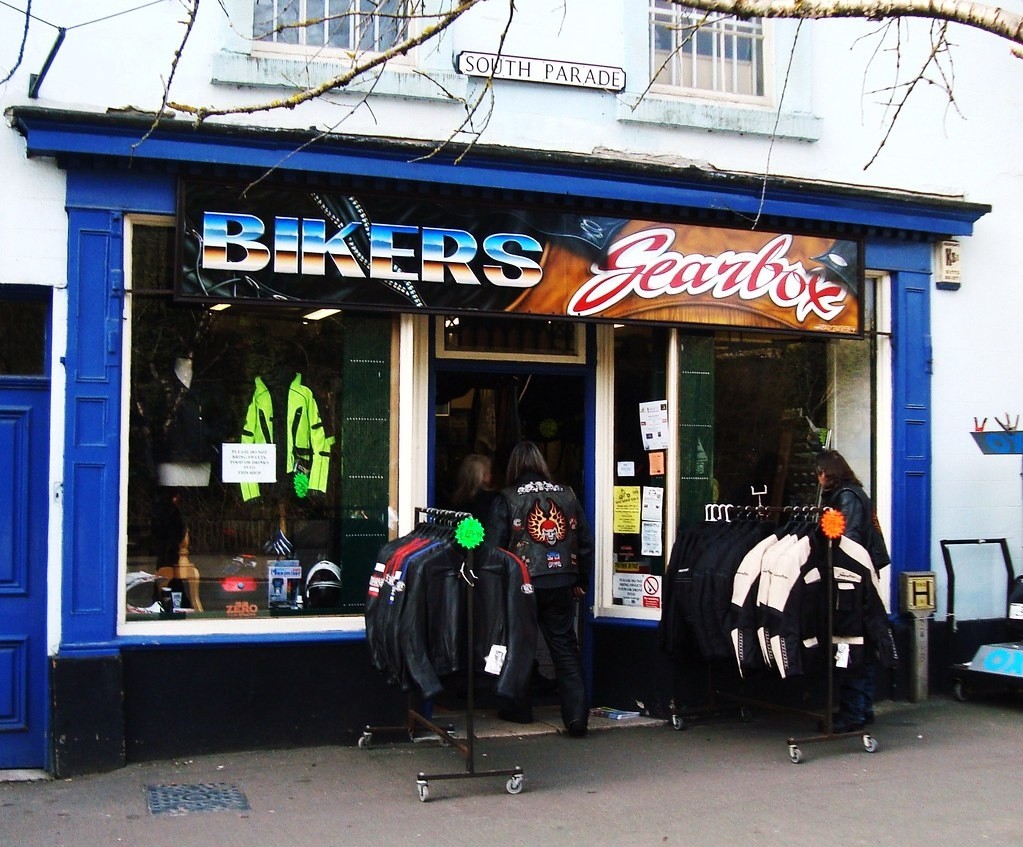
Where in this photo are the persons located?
[480,441,594,738]
[804,448,892,734]
[439,453,500,549]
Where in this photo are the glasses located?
[815,466,826,475]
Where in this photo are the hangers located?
[702,501,840,543]
[409,506,474,547]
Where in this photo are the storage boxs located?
[265,560,302,610]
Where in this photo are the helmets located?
[306,560,341,608]
[126,569,167,614]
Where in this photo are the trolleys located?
[940,538,1022,712]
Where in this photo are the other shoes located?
[818,714,864,733]
[863,711,874,724]
[568,719,587,737]
[498,704,533,723]
